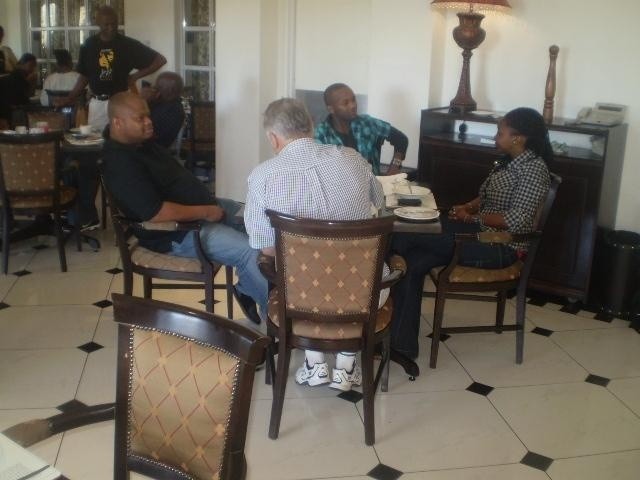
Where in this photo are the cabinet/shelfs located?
[418,106,628,305]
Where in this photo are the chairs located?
[0,89,216,276]
[417,172,561,370]
[264,208,406,446]
[107,295,270,479]
[97,171,233,320]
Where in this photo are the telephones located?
[576,102,626,126]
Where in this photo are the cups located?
[80,125,92,135]
[0,126,41,135]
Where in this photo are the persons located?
[51,6,166,129]
[314,83,409,176]
[55,71,185,234]
[40,47,92,113]
[0,27,18,73]
[0,51,40,118]
[385,107,554,358]
[243,99,392,391]
[102,90,270,326]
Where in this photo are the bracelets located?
[463,213,481,224]
[466,202,473,211]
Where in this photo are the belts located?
[89,93,111,100]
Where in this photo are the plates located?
[396,184,431,199]
[68,127,80,134]
[394,207,440,221]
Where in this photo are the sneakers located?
[230,284,261,324]
[294,357,332,388]
[330,360,362,390]
[49,208,100,234]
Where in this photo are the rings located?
[452,208,456,211]
[452,211,456,216]
[453,216,457,220]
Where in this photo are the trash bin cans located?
[599,230,640,320]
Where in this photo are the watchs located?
[392,158,402,168]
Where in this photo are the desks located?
[272,184,439,378]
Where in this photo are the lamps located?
[430,0,513,114]
